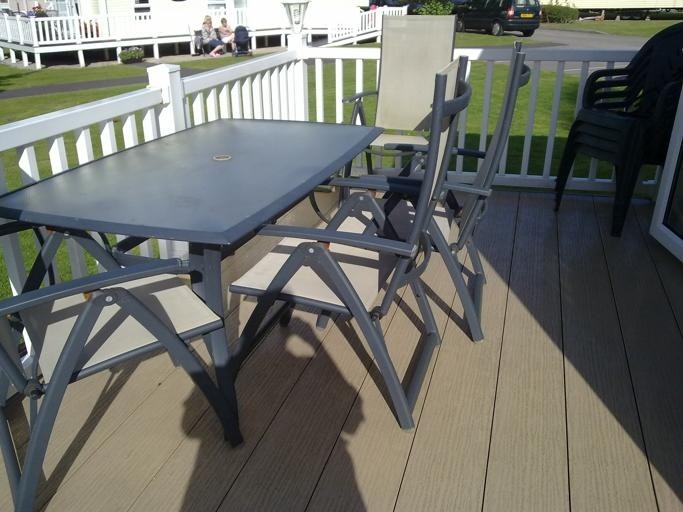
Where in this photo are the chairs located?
[553,22,683,237]
[210,54,473,429]
[332,13,463,213]
[0,227,245,510]
[313,38,531,346]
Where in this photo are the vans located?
[456,0,542,36]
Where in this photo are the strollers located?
[234,26,249,57]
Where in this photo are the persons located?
[35,6,48,42]
[217,18,237,55]
[201,15,224,59]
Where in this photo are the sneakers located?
[208,51,221,57]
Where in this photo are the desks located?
[3,121,385,446]
